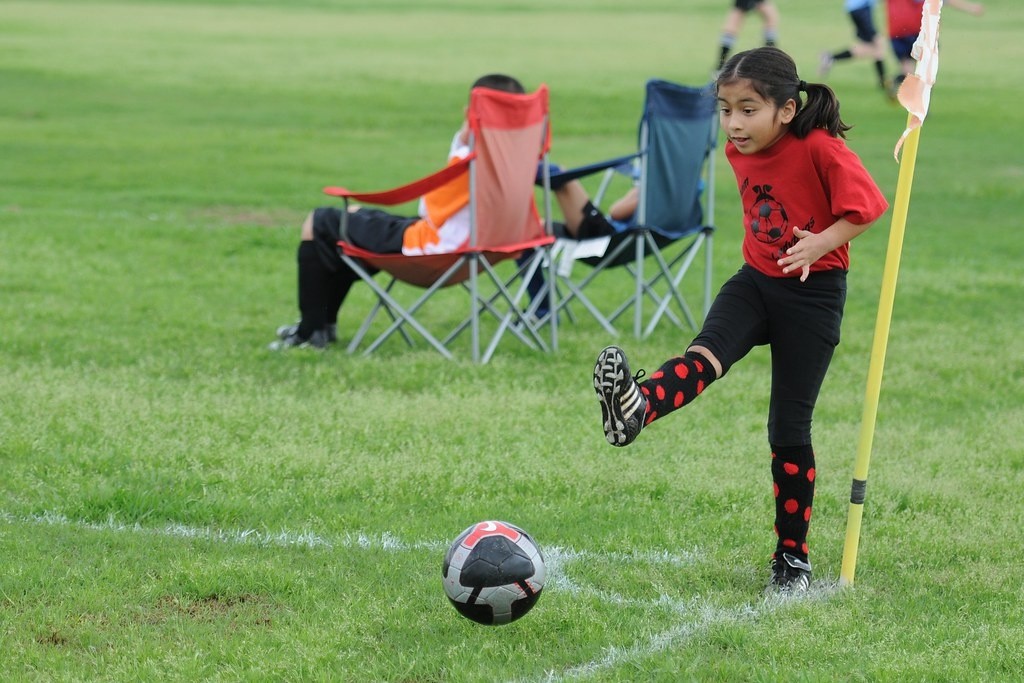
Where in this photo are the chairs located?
[513,81,717,340]
[322,85,561,366]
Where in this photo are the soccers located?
[442,519,546,626]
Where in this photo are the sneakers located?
[765,552,812,600]
[269,322,338,351]
[592,345,648,448]
[818,52,835,83]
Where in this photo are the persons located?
[883,0,983,97]
[268,75,527,352]
[818,0,888,91]
[594,47,890,602]
[710,0,782,83]
[510,154,706,329]
